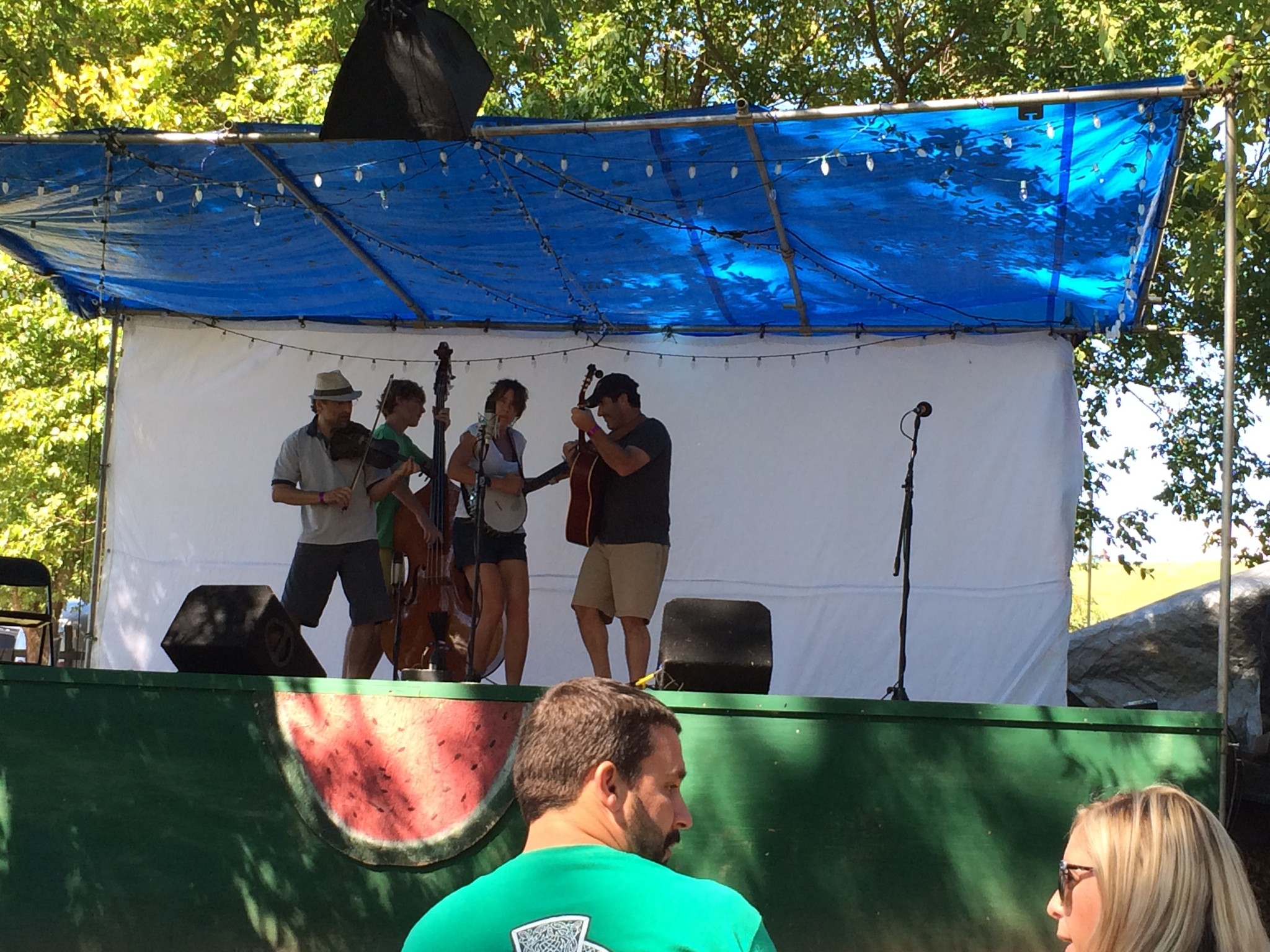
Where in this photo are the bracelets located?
[319,491,325,504]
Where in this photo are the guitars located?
[565,362,604,550]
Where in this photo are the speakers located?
[660,597,774,694]
[162,584,329,677]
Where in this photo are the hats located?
[585,373,639,408]
[309,369,362,401]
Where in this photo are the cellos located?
[380,340,508,683]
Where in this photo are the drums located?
[468,461,567,539]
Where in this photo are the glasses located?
[1056,861,1094,899]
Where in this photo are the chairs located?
[0,553,57,667]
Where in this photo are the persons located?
[447,378,558,685]
[1046,783,1270,952]
[563,372,673,688]
[341,378,443,680]
[400,676,777,952]
[271,370,420,680]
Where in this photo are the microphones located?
[915,401,932,417]
[481,400,499,445]
